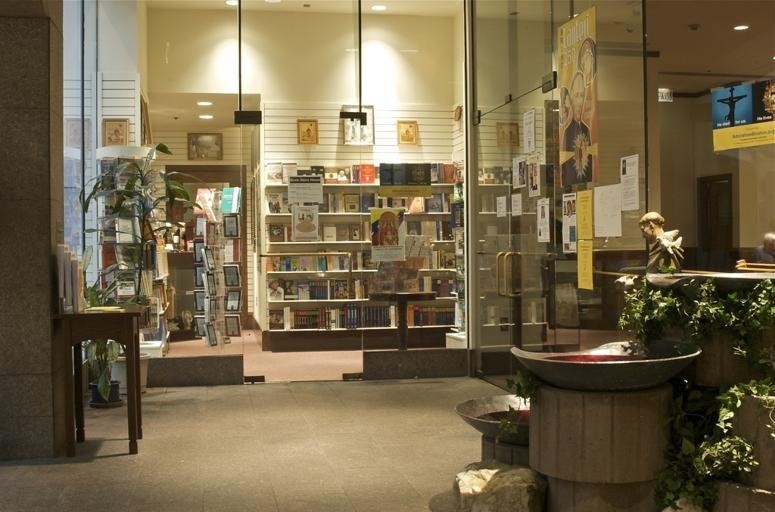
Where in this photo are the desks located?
[58,306,143,455]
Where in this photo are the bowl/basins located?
[454,395,530,443]
[510,337,703,393]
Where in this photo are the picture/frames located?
[100,118,131,148]
[496,122,520,148]
[398,121,418,143]
[297,119,319,146]
[341,104,376,146]
[187,133,223,160]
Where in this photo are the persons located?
[559,73,595,192]
[757,230,775,265]
[636,212,686,272]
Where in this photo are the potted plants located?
[78,143,213,409]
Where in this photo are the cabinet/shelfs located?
[261,160,463,343]
[478,183,556,351]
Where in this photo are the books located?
[54,243,125,315]
[100,155,245,355]
[260,155,468,331]
